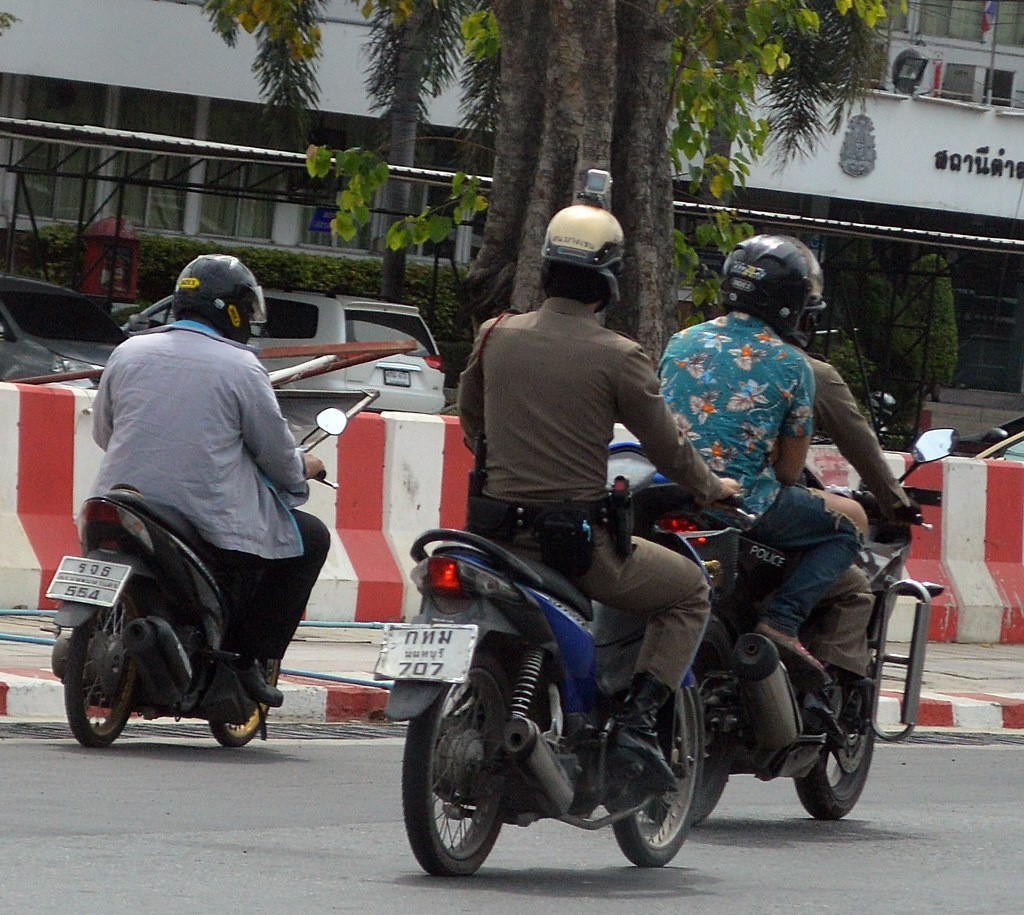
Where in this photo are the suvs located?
[120,282,445,418]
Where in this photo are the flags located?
[981,0,997,32]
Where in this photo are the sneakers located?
[759,617,831,687]
[803,682,833,716]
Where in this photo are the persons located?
[655,233,924,691]
[93,255,331,707]
[458,206,740,793]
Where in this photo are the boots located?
[616,674,681,795]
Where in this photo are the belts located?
[466,496,612,534]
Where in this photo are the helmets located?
[541,200,629,268]
[172,254,257,344]
[722,232,823,349]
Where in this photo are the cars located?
[973,425,1023,466]
[1,273,129,392]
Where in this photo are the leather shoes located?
[219,646,285,710]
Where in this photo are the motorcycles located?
[375,445,756,880]
[30,408,350,752]
[638,426,958,833]
